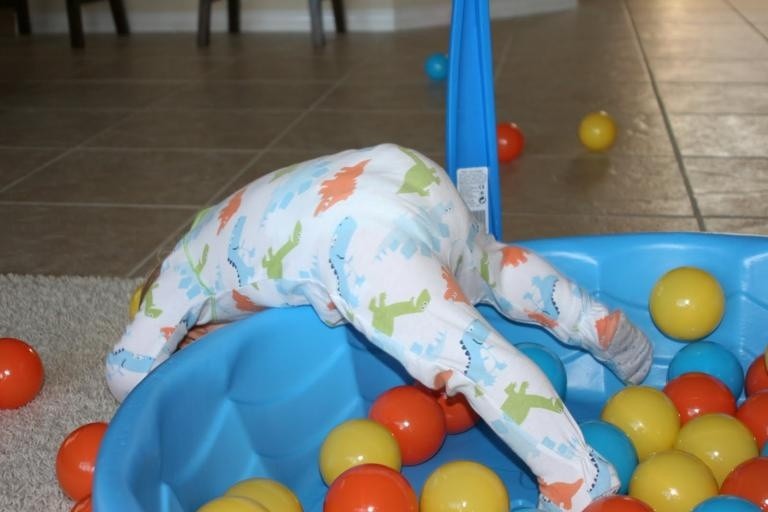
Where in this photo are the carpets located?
[0,272,148,512]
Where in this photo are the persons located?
[105,142,653,512]
